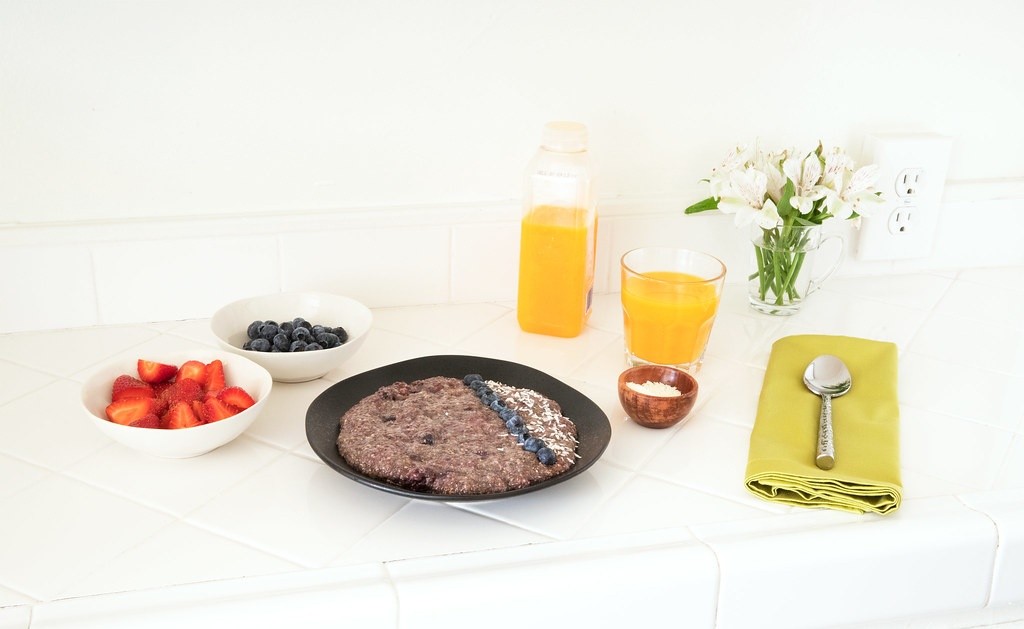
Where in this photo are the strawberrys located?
[105,357,255,430]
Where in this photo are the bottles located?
[517,122,600,338]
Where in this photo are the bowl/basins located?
[618,365,699,429]
[81,350,273,459]
[210,293,373,382]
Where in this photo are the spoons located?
[803,355,852,471]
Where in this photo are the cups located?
[620,248,727,378]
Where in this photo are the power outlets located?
[888,205,924,236]
[896,167,928,200]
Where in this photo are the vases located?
[745,216,846,318]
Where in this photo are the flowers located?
[683,137,887,229]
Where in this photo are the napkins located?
[745,333,904,519]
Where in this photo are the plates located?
[305,355,612,501]
